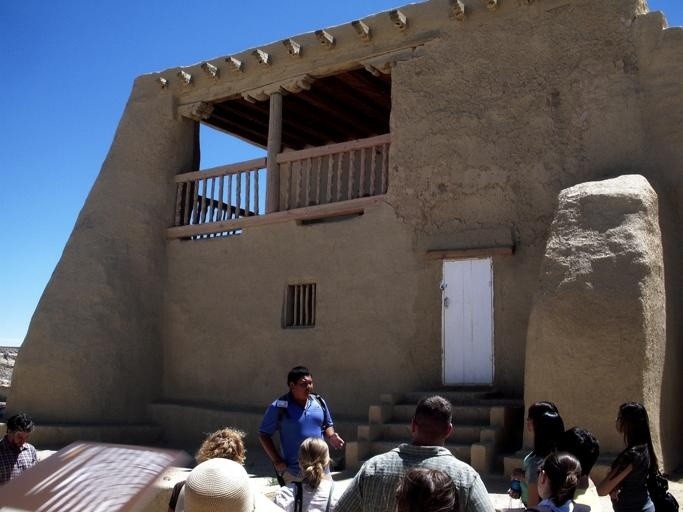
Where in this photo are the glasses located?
[537,470,542,477]
[615,417,622,422]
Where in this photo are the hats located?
[184,457,255,512]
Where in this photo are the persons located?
[259,366,344,483]
[525,451,587,512]
[394,467,457,512]
[273,436,345,512]
[564,426,600,512]
[194,428,247,467]
[519,401,564,509]
[596,402,656,512]
[330,393,496,512]
[0,411,38,487]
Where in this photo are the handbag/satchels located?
[648,469,679,512]
